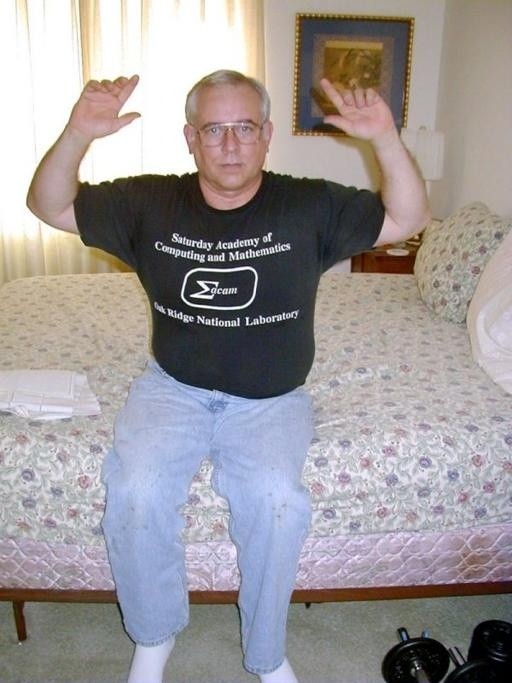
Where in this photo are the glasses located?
[189,122,265,145]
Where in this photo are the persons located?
[26,70,431,683]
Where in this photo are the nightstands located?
[351,240,432,272]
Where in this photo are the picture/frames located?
[293,11,414,139]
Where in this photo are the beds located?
[1,272,512,639]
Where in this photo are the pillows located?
[465,230,512,396]
[412,199,511,323]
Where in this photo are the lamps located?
[401,129,445,244]
